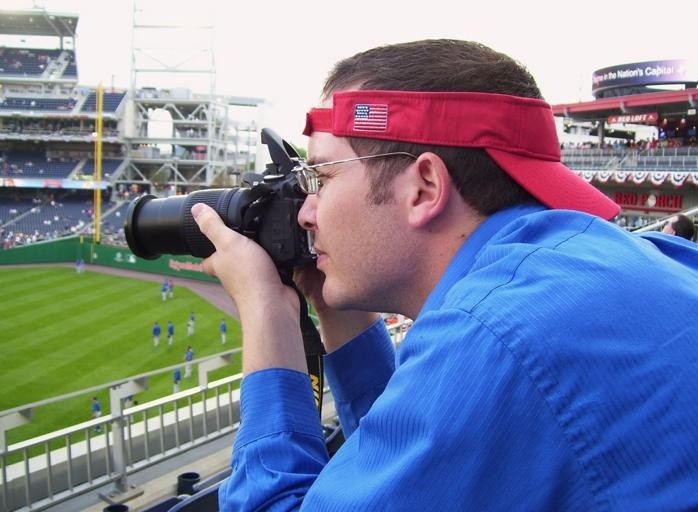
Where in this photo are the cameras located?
[123,127,318,271]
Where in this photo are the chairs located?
[139,416,343,511]
[0,47,130,245]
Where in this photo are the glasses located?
[289,151,413,194]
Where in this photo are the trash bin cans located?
[177,472,200,496]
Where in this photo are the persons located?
[151,321,162,347]
[90,396,102,434]
[660,214,695,241]
[184,347,195,379]
[75,256,85,273]
[168,279,173,299]
[558,124,698,165]
[123,393,135,423]
[609,210,666,233]
[186,311,195,337]
[187,35,698,511]
[161,279,168,301]
[219,318,227,343]
[172,366,182,393]
[1,48,77,76]
[167,321,176,345]
[1,96,149,249]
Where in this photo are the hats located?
[304,88,620,221]
